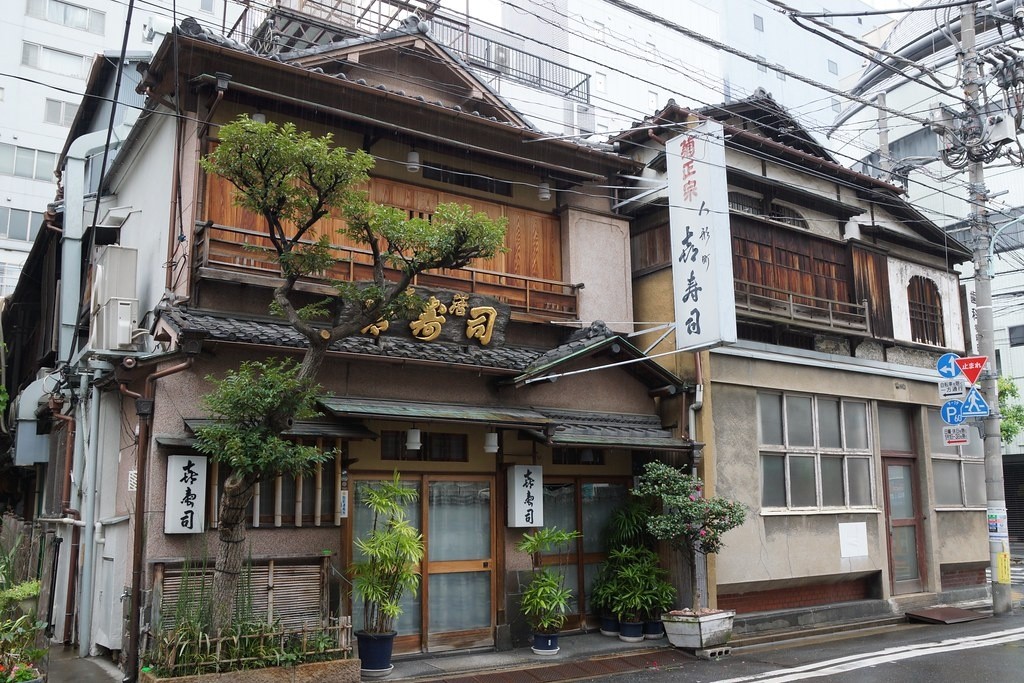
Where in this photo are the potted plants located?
[618,545,654,642]
[630,456,744,646]
[594,565,621,636]
[644,566,678,639]
[348,468,424,677]
[516,526,584,655]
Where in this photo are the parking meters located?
[941,399,966,425]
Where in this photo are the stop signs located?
[954,355,987,385]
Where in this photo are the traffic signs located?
[941,426,971,447]
[937,379,966,399]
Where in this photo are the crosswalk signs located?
[960,386,990,418]
